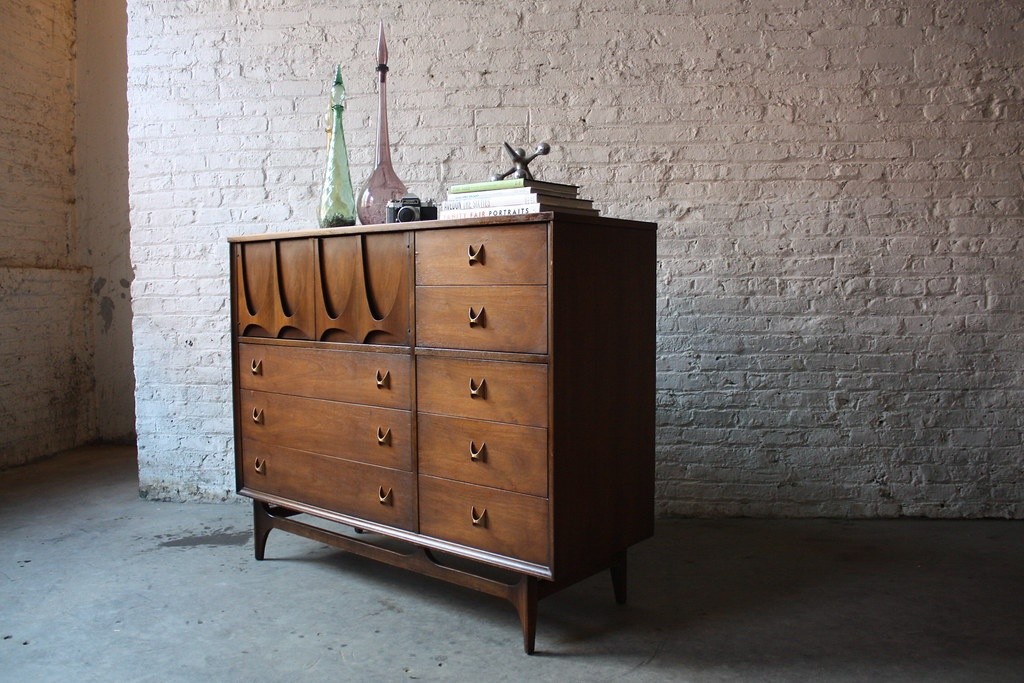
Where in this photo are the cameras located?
[386,193,437,223]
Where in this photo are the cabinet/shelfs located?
[225,210,658,659]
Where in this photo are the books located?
[437,178,601,220]
